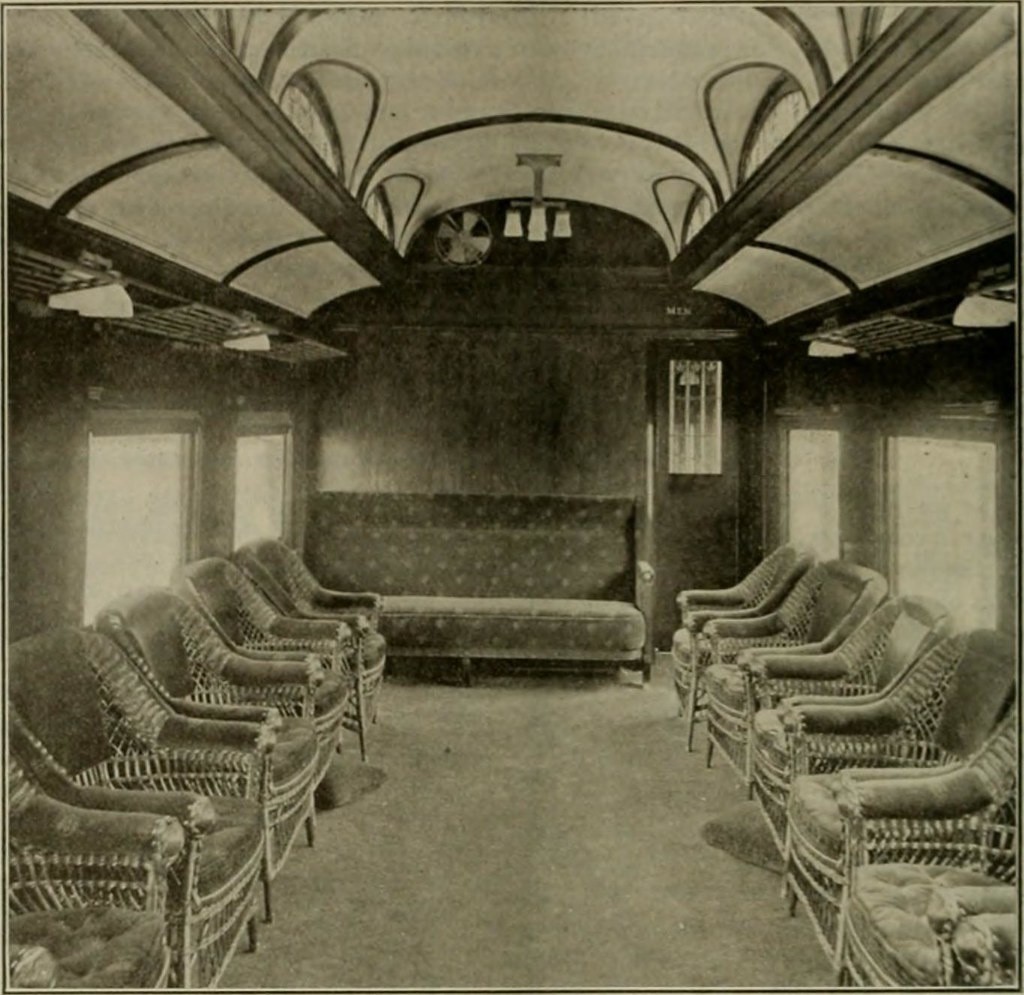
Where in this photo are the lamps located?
[503,153,574,242]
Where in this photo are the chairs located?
[13,537,389,992]
[671,543,1021,991]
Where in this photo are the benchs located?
[304,489,656,684]
